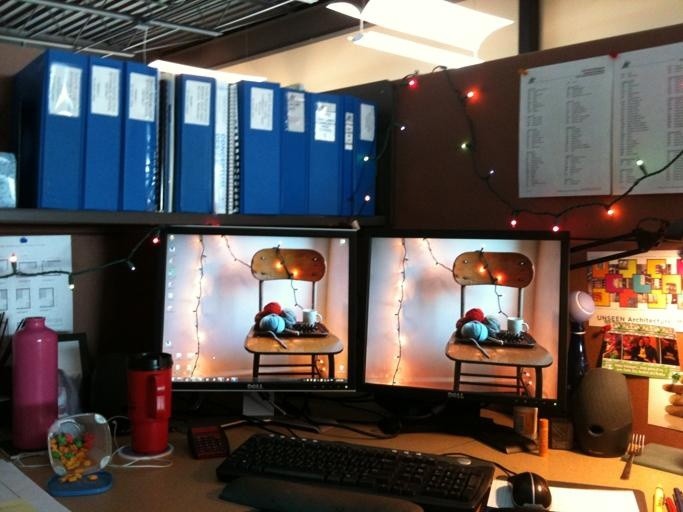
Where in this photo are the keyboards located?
[216,433,496,512]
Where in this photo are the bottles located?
[14,317,58,453]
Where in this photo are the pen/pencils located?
[652,484,683,511]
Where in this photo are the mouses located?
[508,471,552,512]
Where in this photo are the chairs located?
[445,251,552,399]
[244,249,344,379]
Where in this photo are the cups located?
[301,308,322,327]
[124,350,171,454]
[506,316,528,337]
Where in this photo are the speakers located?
[572,368,633,458]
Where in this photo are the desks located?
[0,418,682,511]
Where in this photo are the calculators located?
[188,423,229,458]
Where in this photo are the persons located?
[629,336,657,362]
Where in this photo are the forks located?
[619,431,645,478]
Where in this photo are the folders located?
[14,51,383,216]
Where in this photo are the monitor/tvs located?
[153,224,358,434]
[356,227,571,454]
